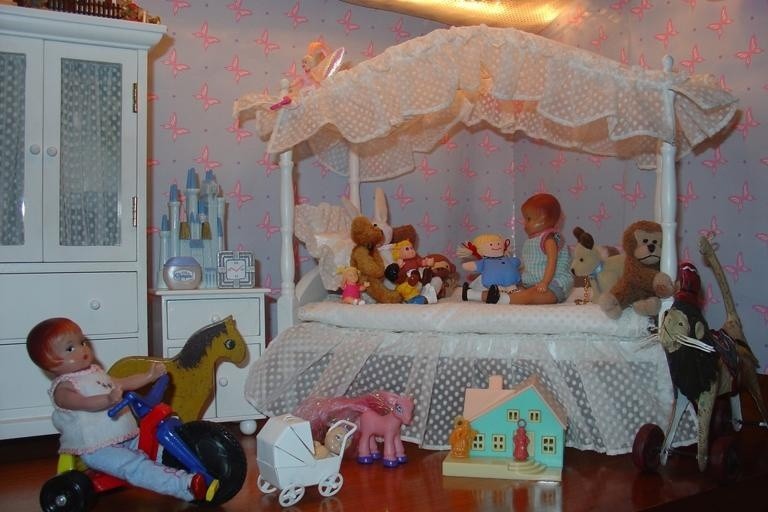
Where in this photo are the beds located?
[277,25,681,416]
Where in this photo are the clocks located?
[216,251,256,290]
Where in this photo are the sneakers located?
[189,474,206,501]
[487,284,499,304]
[462,282,471,301]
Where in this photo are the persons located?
[462,192,573,305]
[25,315,248,512]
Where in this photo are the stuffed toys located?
[601,219,675,320]
[457,233,519,289]
[570,226,624,306]
[385,240,435,284]
[633,233,768,479]
[335,264,370,306]
[424,254,456,299]
[406,276,442,305]
[350,216,402,304]
[341,187,423,290]
[396,270,428,305]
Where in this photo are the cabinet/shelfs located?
[1,4,168,442]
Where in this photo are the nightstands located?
[146,288,273,436]
